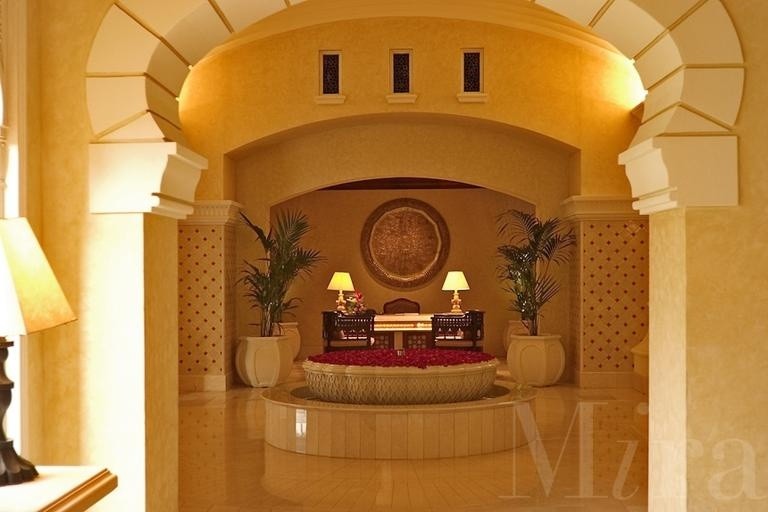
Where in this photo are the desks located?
[0,465,118,512]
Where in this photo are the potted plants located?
[493,209,578,388]
[234,207,328,388]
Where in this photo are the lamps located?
[1,218,78,486]
[442,270,470,312]
[327,272,355,311]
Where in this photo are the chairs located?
[430,309,485,350]
[322,310,375,353]
